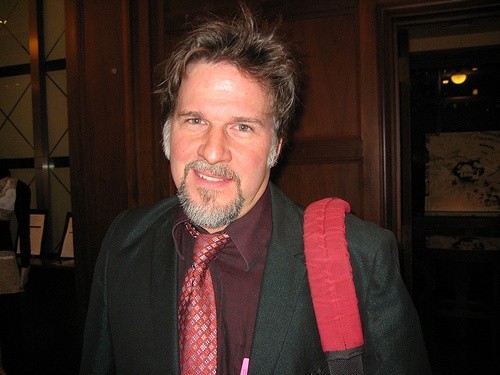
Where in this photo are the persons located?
[77,0,426,374]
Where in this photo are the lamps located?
[438,67,479,104]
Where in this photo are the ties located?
[176,221,232,375]
